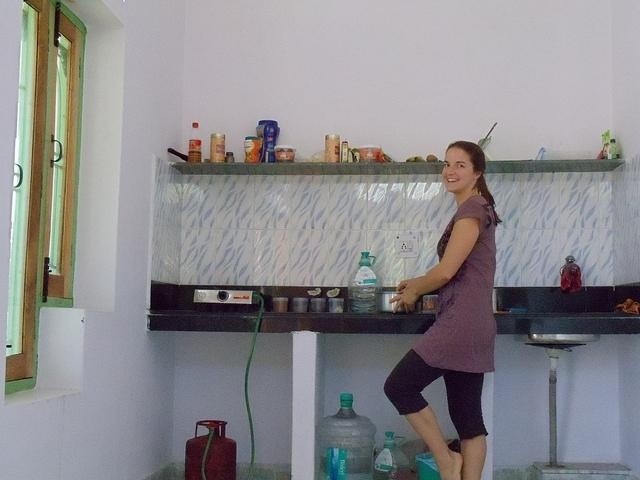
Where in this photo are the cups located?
[273,297,343,314]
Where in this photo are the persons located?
[383,141,503,480]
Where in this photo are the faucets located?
[561,255,578,276]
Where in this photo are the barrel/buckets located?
[315,393,377,480]
[374,431,409,476]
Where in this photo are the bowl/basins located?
[377,291,415,313]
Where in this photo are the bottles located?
[187,122,201,164]
[369,430,409,480]
[608,139,619,160]
[262,122,278,162]
[559,257,583,293]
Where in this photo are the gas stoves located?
[190,287,262,310]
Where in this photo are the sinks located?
[529,312,601,344]
